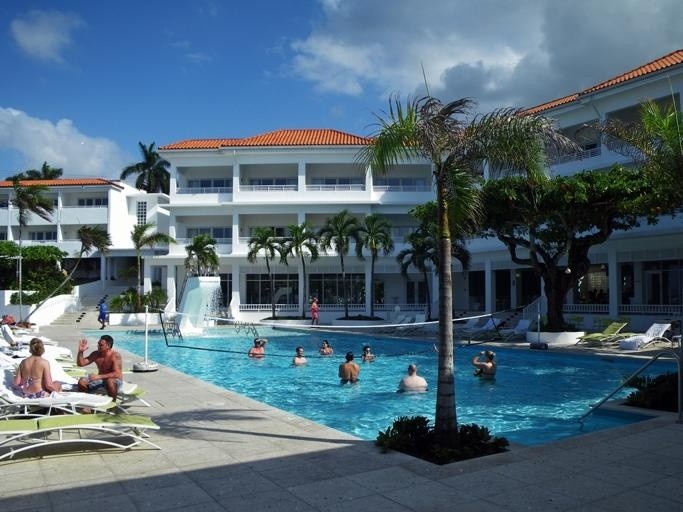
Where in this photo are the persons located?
[0,315,36,329]
[588,288,609,304]
[96,299,107,329]
[292,347,307,365]
[472,349,497,378]
[320,339,334,356]
[309,297,321,326]
[13,336,62,397]
[338,351,359,383]
[360,345,375,362]
[74,334,123,414]
[247,338,268,359]
[399,364,428,392]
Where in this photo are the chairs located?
[574,321,674,351]
[377,313,426,338]
[0,323,57,348]
[453,316,533,343]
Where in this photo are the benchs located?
[0,346,161,460]
